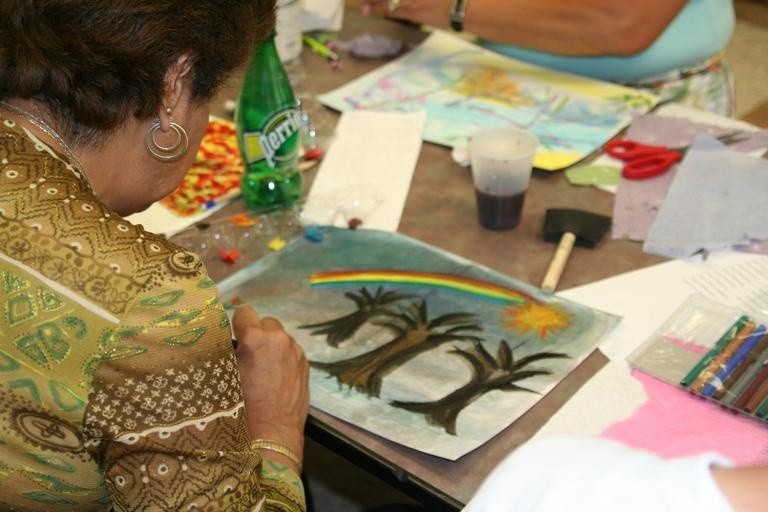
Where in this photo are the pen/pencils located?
[303,34,340,61]
[681,316,768,422]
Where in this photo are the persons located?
[370,0,734,120]
[1,0,309,512]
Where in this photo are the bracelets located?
[448,0,468,32]
[250,439,304,474]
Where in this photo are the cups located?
[464,121,541,232]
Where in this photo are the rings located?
[387,1,400,13]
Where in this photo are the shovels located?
[541,208,611,294]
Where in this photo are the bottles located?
[235,19,307,214]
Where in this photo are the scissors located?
[604,131,751,179]
